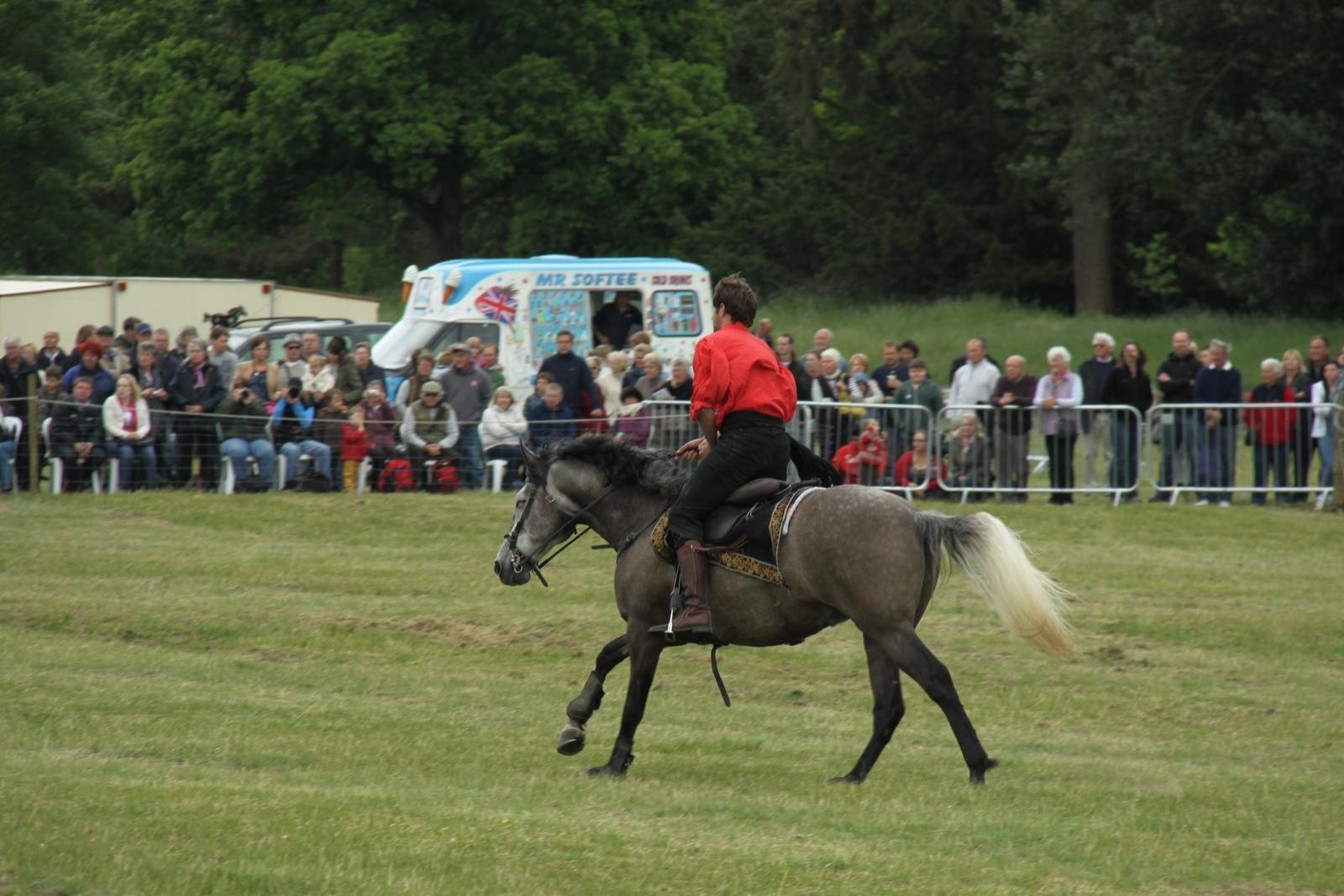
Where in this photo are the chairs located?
[0,417,506,494]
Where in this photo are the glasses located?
[424,392,439,398]
[285,344,301,350]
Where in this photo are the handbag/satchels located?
[125,436,153,448]
[1246,428,1258,446]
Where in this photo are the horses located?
[491,430,1080,788]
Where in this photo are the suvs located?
[217,317,393,378]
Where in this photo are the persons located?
[647,275,796,634]
[595,291,641,349]
[0,317,692,492]
[759,319,1344,509]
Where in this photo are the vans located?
[366,251,712,404]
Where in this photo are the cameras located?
[288,389,299,399]
[241,391,249,398]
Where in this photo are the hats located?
[136,323,150,333]
[419,381,443,393]
[449,344,473,354]
[97,326,114,338]
[283,334,302,348]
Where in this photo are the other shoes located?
[1196,499,1209,506]
[1218,499,1231,507]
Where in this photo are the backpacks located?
[430,460,461,493]
[376,458,415,494]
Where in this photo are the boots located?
[647,540,712,640]
